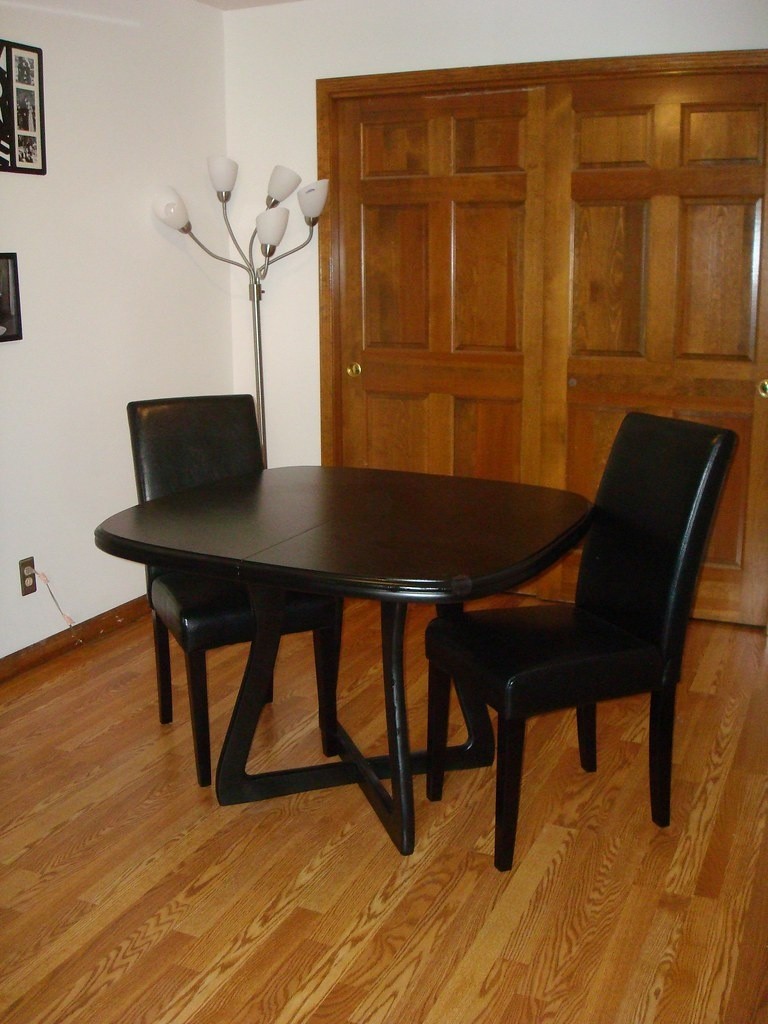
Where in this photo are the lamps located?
[153,155,329,468]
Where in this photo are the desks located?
[94,466,592,856]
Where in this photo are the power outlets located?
[19,557,36,596]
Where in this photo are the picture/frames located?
[0,39,46,175]
[0,253,22,342]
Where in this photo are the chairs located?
[425,410,739,872]
[127,394,344,787]
